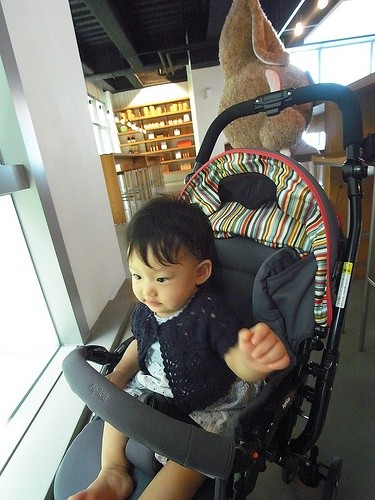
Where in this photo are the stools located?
[117,162,163,216]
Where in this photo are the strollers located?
[52,81,375,500]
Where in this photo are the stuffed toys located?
[214,0,318,163]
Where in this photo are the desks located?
[100,153,166,224]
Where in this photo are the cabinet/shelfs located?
[311,72,375,280]
[113,97,196,174]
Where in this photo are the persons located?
[61,192,290,500]
